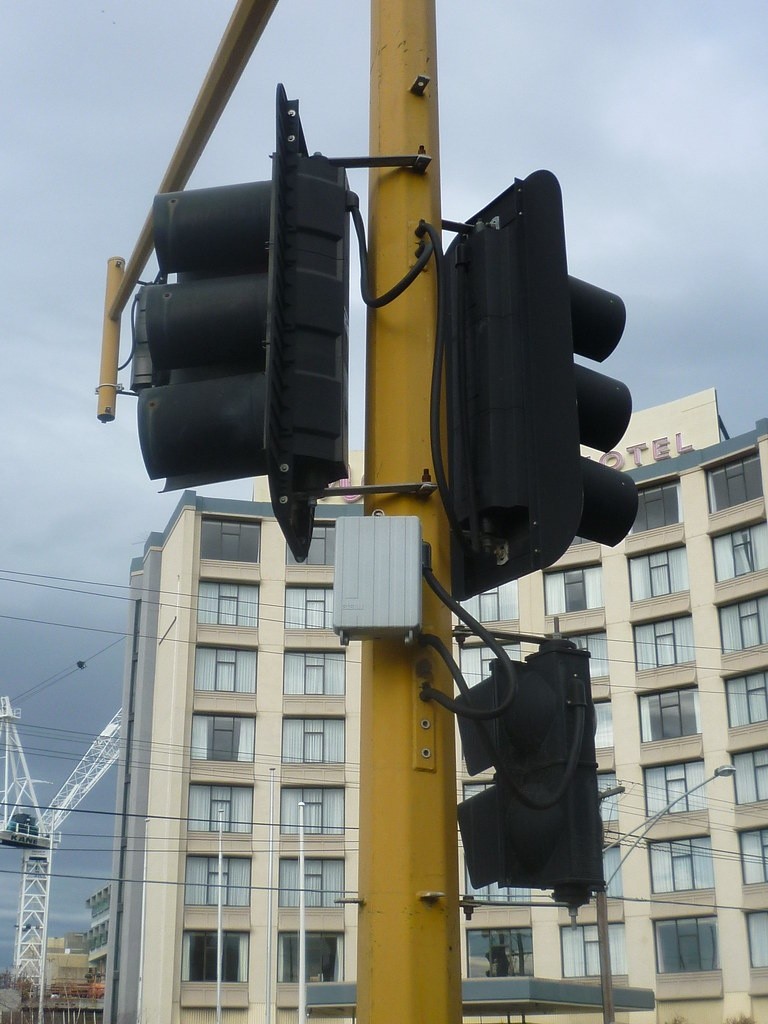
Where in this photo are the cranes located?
[0,696,121,1024]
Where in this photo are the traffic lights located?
[454,640,607,913]
[435,170,638,598]
[136,83,356,564]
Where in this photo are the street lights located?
[595,765,737,1024]
[137,819,148,1024]
[297,802,306,1024]
[217,809,224,1024]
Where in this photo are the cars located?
[50,992,60,998]
